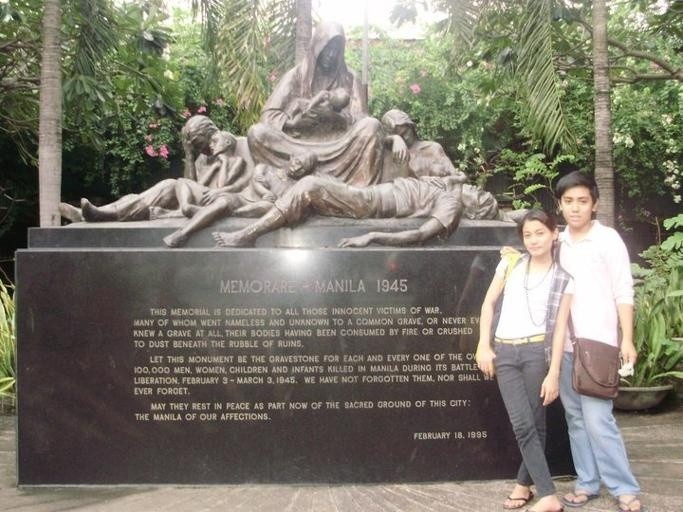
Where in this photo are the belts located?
[492,334,547,345]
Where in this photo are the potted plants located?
[614,270,682,411]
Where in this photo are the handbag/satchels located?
[571,337,622,399]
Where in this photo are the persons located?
[474,210,575,512]
[57,22,498,246]
[498,170,644,512]
[454,252,498,327]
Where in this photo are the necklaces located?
[523,254,555,290]
[525,256,554,327]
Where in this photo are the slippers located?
[503,490,533,509]
[562,489,600,507]
[618,496,642,512]
[525,496,564,512]
